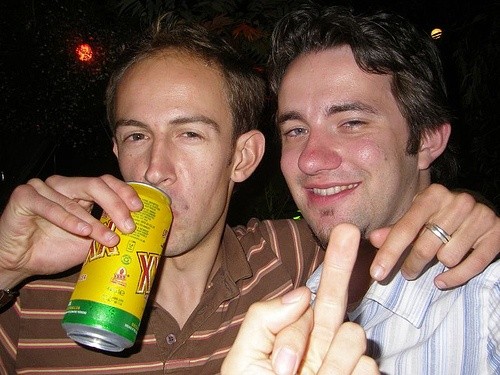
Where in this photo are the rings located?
[426,222,451,244]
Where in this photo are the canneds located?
[60,181,174,352]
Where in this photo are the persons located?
[267,3,500,375]
[0,13,500,374]
